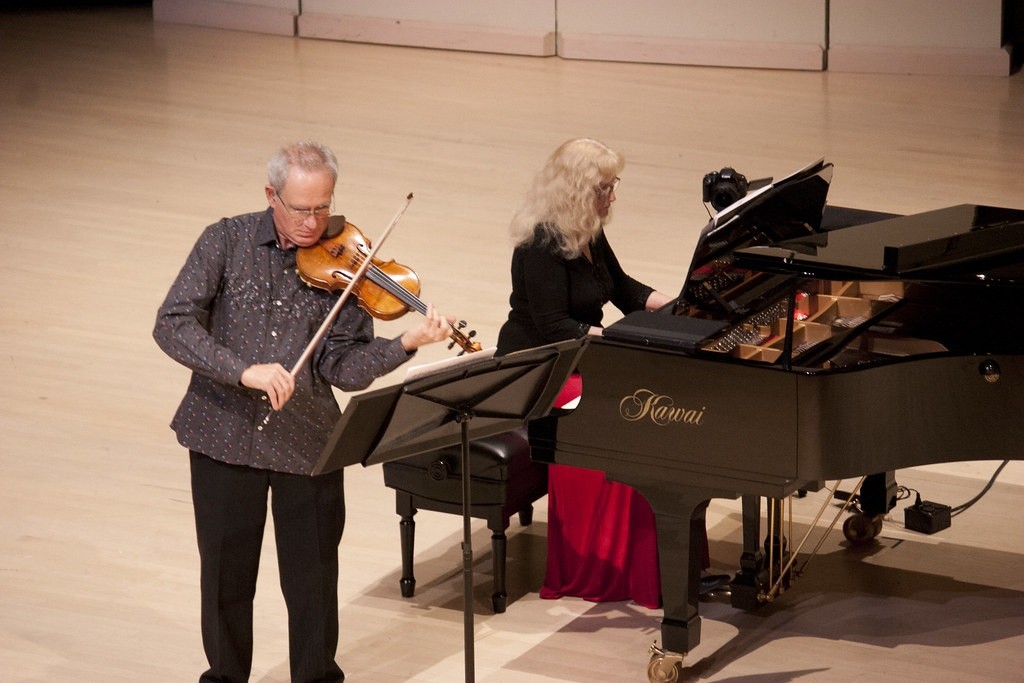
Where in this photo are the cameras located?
[701,166,748,211]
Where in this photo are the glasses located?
[275,189,336,221]
[595,176,620,201]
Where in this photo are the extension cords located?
[905,501,952,535]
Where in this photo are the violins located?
[294,213,482,356]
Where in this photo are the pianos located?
[550,154,1021,682]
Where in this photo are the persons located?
[492,137,731,599]
[151,140,456,683]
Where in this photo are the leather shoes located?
[699,574,730,595]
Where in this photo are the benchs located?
[382,422,558,613]
